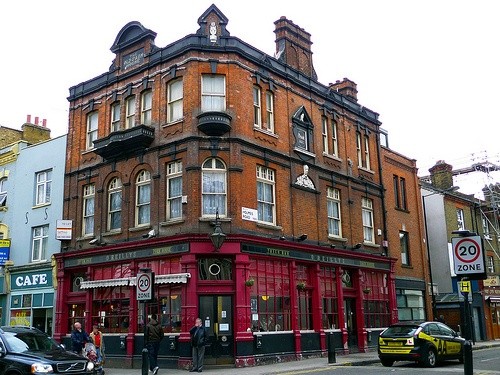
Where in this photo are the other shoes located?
[190,368,201,372]
[152,366,159,374]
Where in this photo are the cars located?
[378,321,471,368]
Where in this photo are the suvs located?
[0,326,96,375]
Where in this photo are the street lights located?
[423,186,460,314]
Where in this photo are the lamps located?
[351,243,362,250]
[296,234,307,242]
[209,208,226,249]
[329,243,336,249]
[89,238,102,247]
[280,235,286,241]
[141,233,149,239]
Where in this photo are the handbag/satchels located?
[98,350,106,366]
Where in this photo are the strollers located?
[81,343,105,375]
